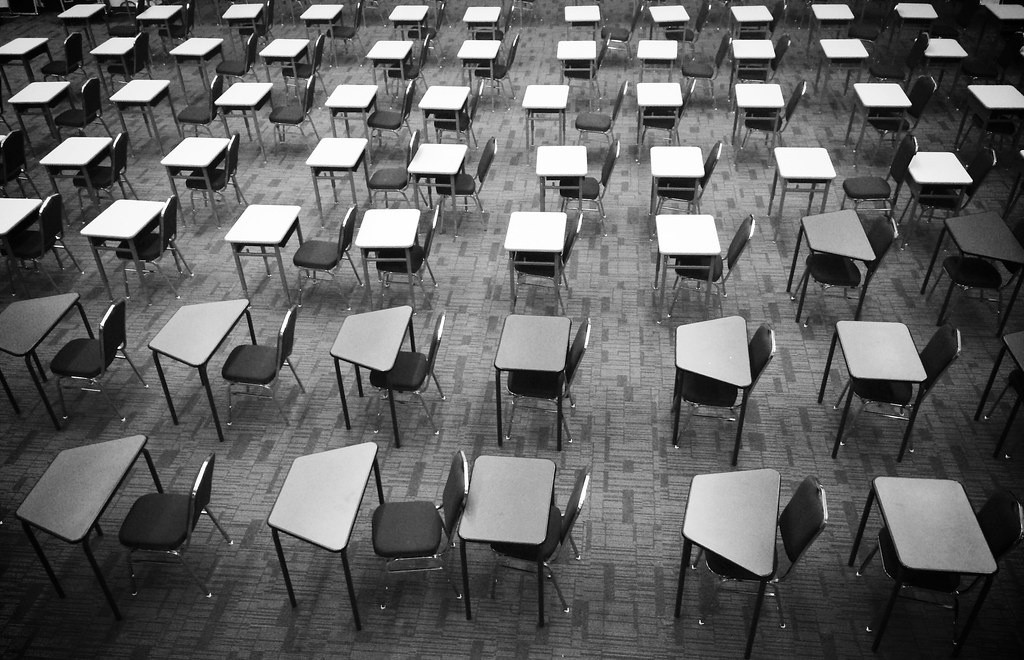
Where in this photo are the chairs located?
[0,1,1023,459]
[372,450,469,608]
[692,475,828,629]
[118,452,232,599]
[855,490,1024,646]
[490,465,591,614]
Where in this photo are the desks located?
[974,330,1024,458]
[267,441,385,633]
[457,454,557,628]
[0,293,95,431]
[148,298,257,442]
[672,315,752,467]
[15,434,164,622]
[818,321,928,463]
[848,476,1001,660]
[0,0,1024,335]
[674,468,781,660]
[330,305,416,447]
[494,314,572,451]
